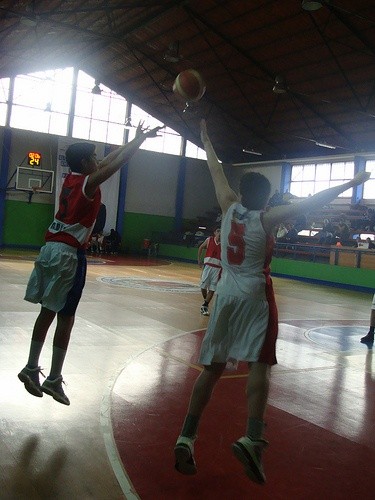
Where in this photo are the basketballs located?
[172,69,206,102]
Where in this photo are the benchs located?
[177,197,375,257]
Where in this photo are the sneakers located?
[18,366,45,397]
[172,435,197,476]
[231,437,271,486]
[41,375,70,405]
[361,332,374,341]
[200,299,209,315]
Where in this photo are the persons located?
[16,122,161,407]
[172,117,375,484]
